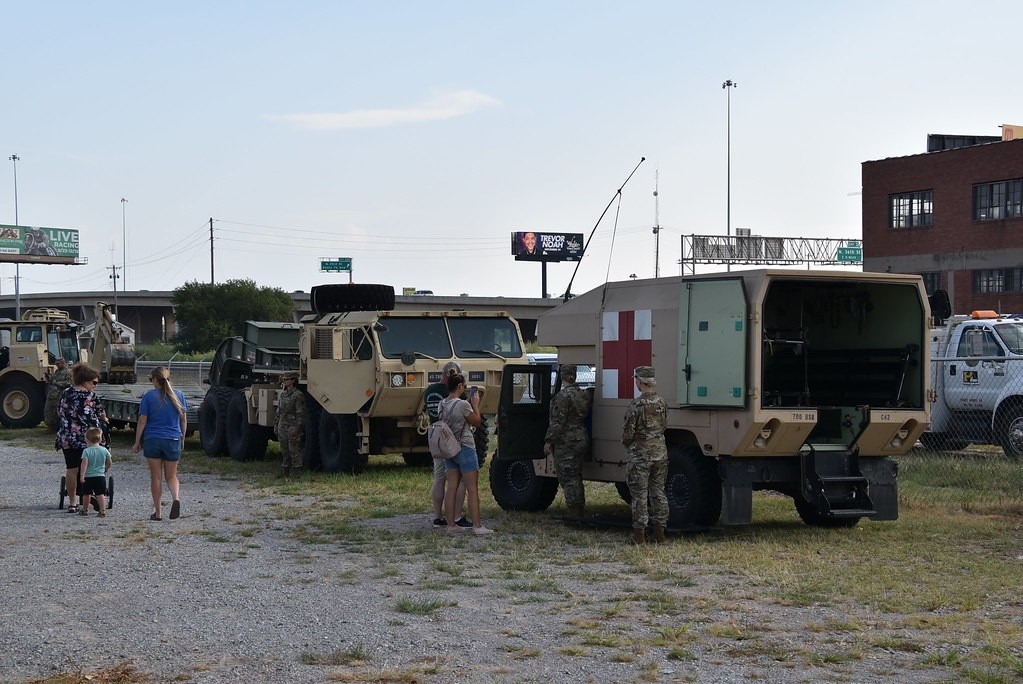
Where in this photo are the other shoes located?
[454,517,473,529]
[97,510,105,517]
[276,466,289,478]
[291,466,302,479]
[625,529,645,545]
[644,526,666,543]
[433,518,448,527]
[553,506,584,520]
[448,525,465,532]
[79,508,88,516]
[471,525,494,534]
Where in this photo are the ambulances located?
[489,155,935,536]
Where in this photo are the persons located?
[520,232,542,255]
[55,363,111,513]
[25,229,58,256]
[79,427,111,518]
[131,366,187,521]
[544,365,591,526]
[621,366,669,546]
[274,372,305,479]
[42,357,72,435]
[424,362,494,534]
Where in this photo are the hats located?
[282,372,300,379]
[55,357,65,363]
[632,366,656,378]
[443,362,462,373]
[561,365,577,371]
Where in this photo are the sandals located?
[90,495,100,511]
[68,504,80,513]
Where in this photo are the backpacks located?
[428,398,466,459]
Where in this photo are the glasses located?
[92,380,97,385]
[149,377,154,382]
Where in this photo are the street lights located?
[121,198,129,291]
[9,154,21,320]
[723,80,738,270]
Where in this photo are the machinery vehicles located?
[0,301,139,429]
[198,283,530,475]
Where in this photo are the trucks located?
[917,310,1023,462]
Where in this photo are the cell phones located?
[471,386,478,396]
[44,373,50,379]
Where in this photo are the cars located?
[412,290,434,296]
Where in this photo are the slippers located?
[169,500,180,519]
[149,512,162,521]
